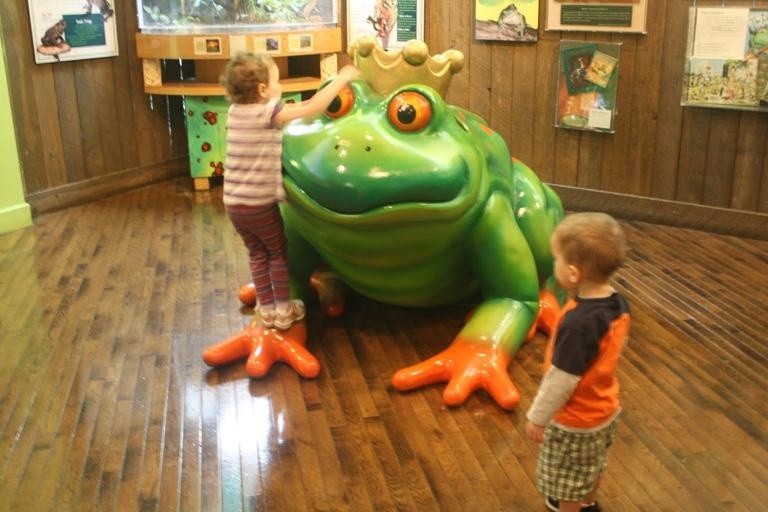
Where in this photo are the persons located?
[218,50,362,332]
[524,210,634,511]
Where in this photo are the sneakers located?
[545,495,600,512]
[254,300,306,330]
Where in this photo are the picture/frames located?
[26,0,120,65]
